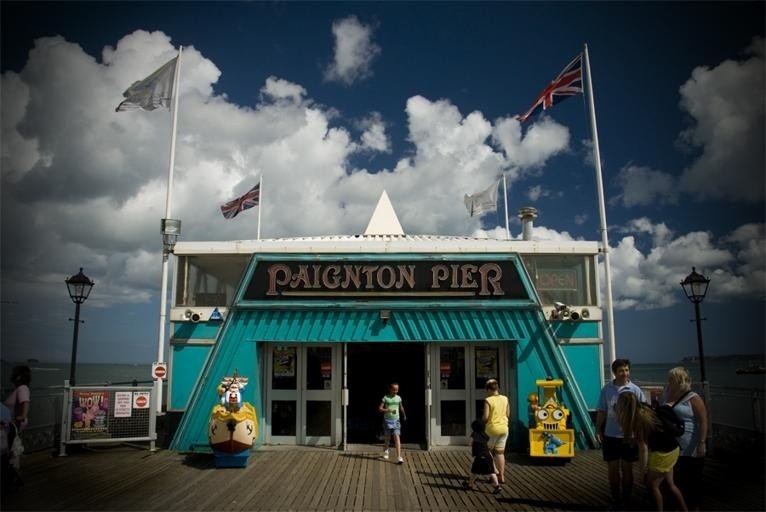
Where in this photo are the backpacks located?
[638,399,686,437]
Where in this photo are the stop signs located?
[150,362,168,379]
[134,390,148,410]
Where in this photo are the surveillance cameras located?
[554,301,567,311]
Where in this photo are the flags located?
[516,52,583,142]
[219,182,259,221]
[463,177,499,218]
[113,54,180,116]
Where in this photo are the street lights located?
[61,266,95,385]
[678,265,711,380]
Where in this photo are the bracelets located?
[700,439,706,442]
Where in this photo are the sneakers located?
[395,456,404,464]
[462,479,473,491]
[383,447,389,459]
[492,485,503,494]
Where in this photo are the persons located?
[481,378,510,485]
[6,362,33,463]
[0,400,9,503]
[614,390,689,511]
[593,357,647,508]
[662,366,709,500]
[378,381,407,464]
[461,419,504,494]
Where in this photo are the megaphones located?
[560,308,570,320]
[580,308,590,320]
[182,309,193,321]
